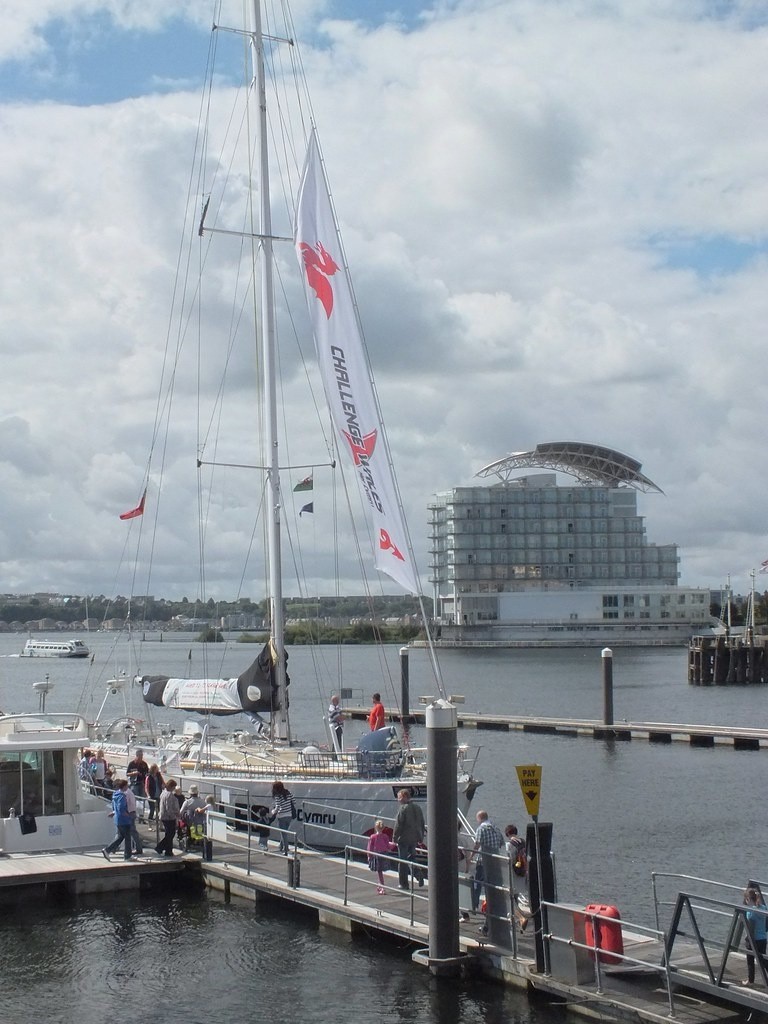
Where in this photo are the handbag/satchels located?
[291,801,297,819]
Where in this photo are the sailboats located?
[23,0,484,866]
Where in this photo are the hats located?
[188,784,199,795]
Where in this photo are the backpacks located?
[508,837,527,877]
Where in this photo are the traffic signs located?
[514,765,543,816]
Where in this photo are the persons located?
[145,764,165,831]
[469,810,528,934]
[741,888,768,986]
[328,695,345,762]
[272,782,296,855]
[125,750,148,824]
[367,693,385,731]
[101,779,143,862]
[393,789,425,889]
[155,779,215,857]
[79,749,113,800]
[251,808,277,851]
[457,820,470,923]
[368,821,397,895]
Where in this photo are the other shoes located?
[459,916,470,922]
[132,849,143,855]
[742,980,754,987]
[520,917,529,934]
[377,886,386,895]
[283,854,287,856]
[332,756,338,762]
[418,876,424,887]
[101,848,110,862]
[259,842,263,847]
[124,856,137,862]
[278,845,282,851]
[263,847,268,851]
[154,847,162,854]
[165,852,174,856]
[398,884,409,890]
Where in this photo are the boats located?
[20,640,90,657]
[0,672,133,856]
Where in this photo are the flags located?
[293,474,313,492]
[299,502,314,516]
